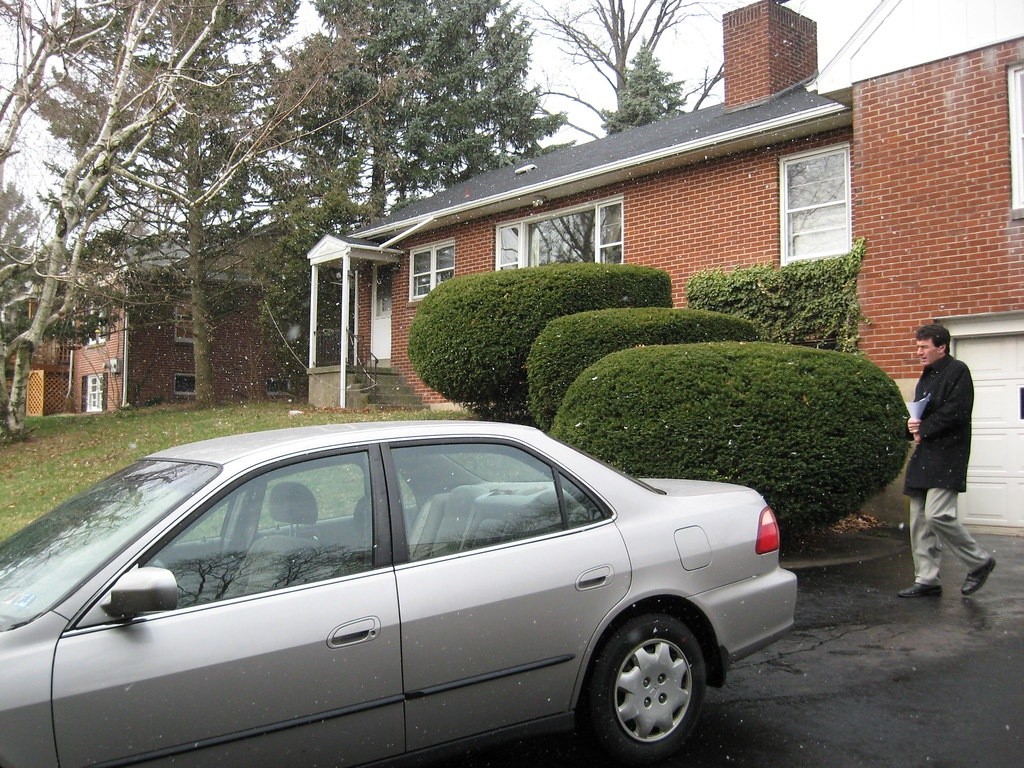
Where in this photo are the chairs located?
[218,481,325,600]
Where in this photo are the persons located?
[897,324,996,598]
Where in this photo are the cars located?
[0,418,799,768]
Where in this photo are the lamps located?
[530,195,546,208]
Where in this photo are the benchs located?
[406,486,586,564]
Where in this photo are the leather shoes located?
[897,581,942,598]
[961,557,996,595]
